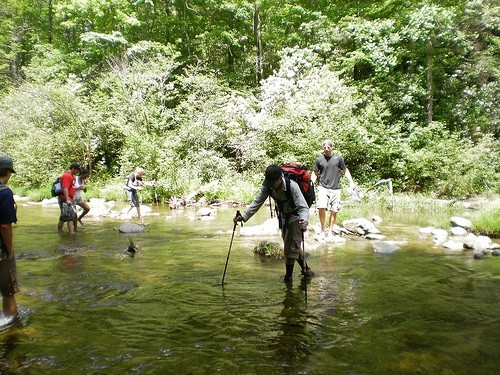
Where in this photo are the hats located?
[262,164,281,187]
[71,163,80,170]
[0,156,16,173]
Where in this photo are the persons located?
[124,168,145,218]
[0,156,20,315]
[313,139,345,240]
[58,164,80,234]
[233,164,315,289]
[74,167,90,231]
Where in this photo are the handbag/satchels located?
[61,202,77,221]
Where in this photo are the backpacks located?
[50,171,73,197]
[280,163,316,208]
